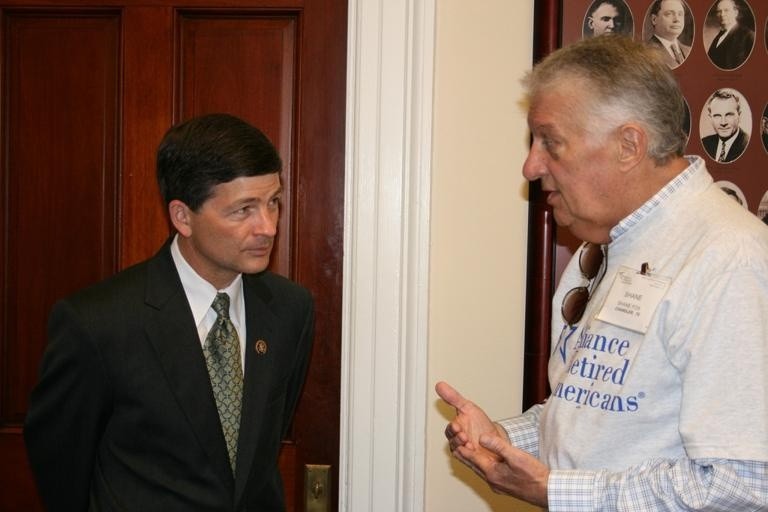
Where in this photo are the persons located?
[21,112,318,512]
[436,34,768,512]
[582,0,768,223]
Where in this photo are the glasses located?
[560,241,609,324]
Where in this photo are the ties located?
[201,291,244,479]
[718,141,727,162]
[671,43,683,64]
